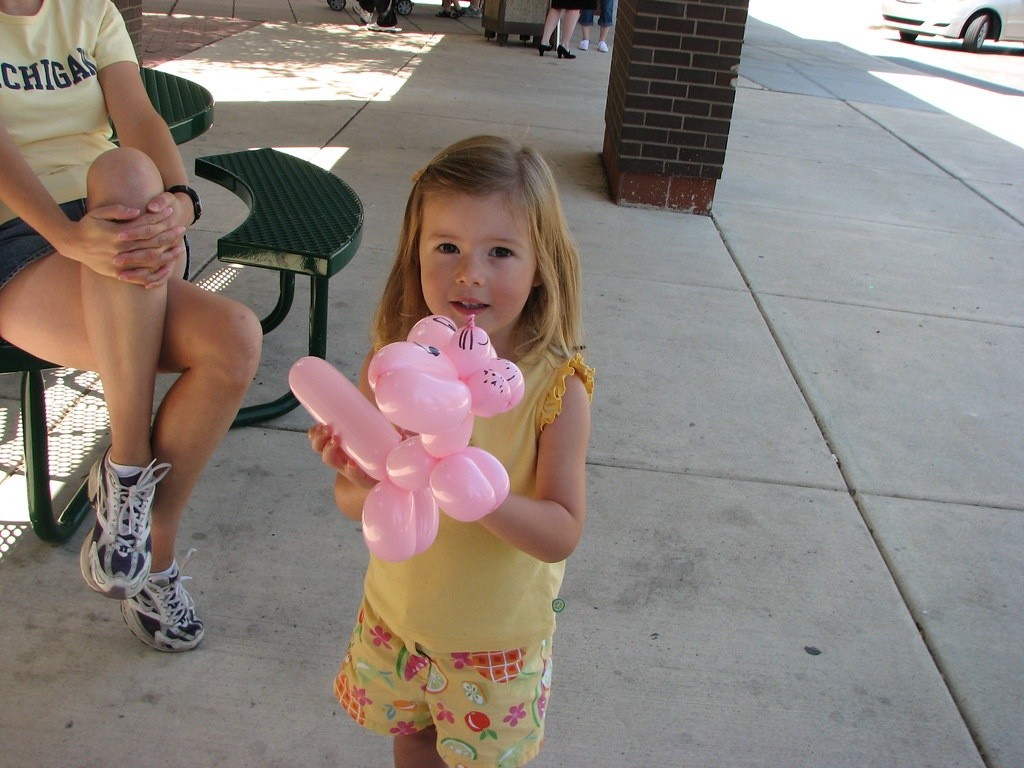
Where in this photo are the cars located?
[880,0,1024,54]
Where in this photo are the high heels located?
[557,45,576,58]
[538,44,552,56]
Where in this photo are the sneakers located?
[576,40,589,50]
[352,3,373,24]
[597,41,608,51]
[366,22,395,32]
[80,444,172,600]
[120,548,205,652]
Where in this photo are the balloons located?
[287,313,525,559]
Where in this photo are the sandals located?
[450,7,465,18]
[435,9,451,18]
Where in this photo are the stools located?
[0,234,189,543]
[194,149,369,429]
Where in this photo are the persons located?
[537,0,597,59]
[578,0,615,52]
[353,0,397,32]
[435,0,482,19]
[307,135,595,768]
[1,0,263,651]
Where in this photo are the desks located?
[103,66,214,152]
